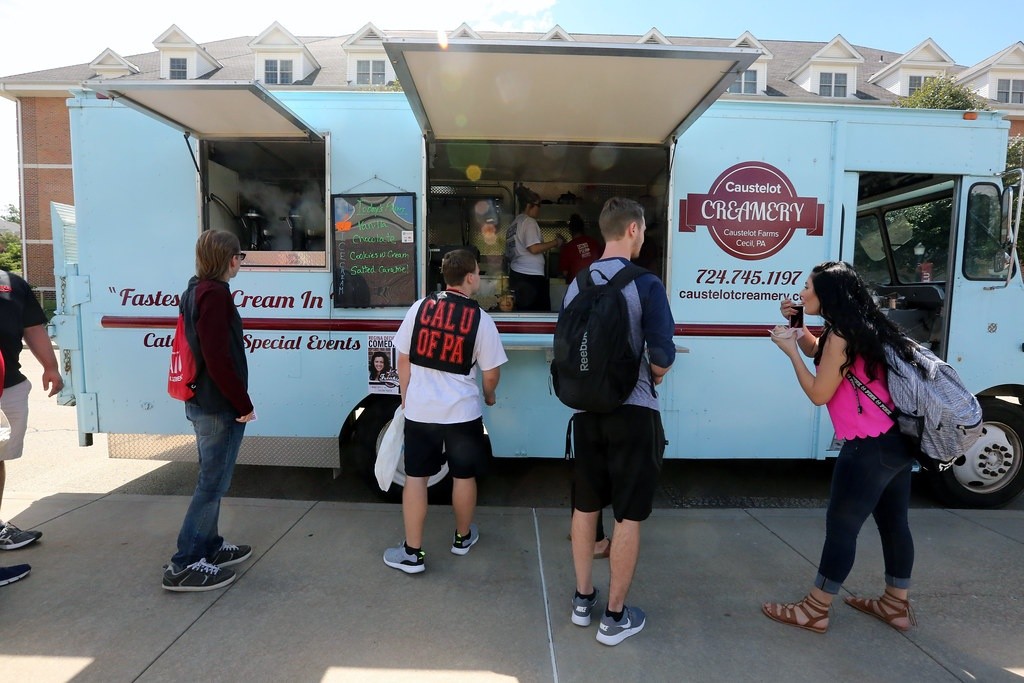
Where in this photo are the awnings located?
[89,78,325,200]
[382,37,761,207]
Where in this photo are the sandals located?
[762,594,838,633]
[843,589,918,631]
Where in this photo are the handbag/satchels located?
[374,404,405,493]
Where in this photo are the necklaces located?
[447,289,468,297]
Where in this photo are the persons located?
[370,352,392,382]
[761,261,915,633]
[0,246,65,585]
[162,230,256,591]
[565,197,676,645]
[506,186,563,314]
[384,250,508,572]
[560,214,602,278]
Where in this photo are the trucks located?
[46,38,1023,512]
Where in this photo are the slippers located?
[593,537,611,558]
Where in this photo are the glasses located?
[231,252,246,261]
[530,202,541,208]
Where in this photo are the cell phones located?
[789,305,805,331]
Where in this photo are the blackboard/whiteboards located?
[330,191,418,308]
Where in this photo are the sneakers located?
[571,587,600,627]
[0,564,31,586]
[206,541,251,568]
[451,522,480,555]
[596,604,646,647]
[161,556,236,592]
[383,540,425,573]
[0,522,41,549]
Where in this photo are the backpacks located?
[817,323,983,472]
[549,263,649,412]
[166,286,204,400]
